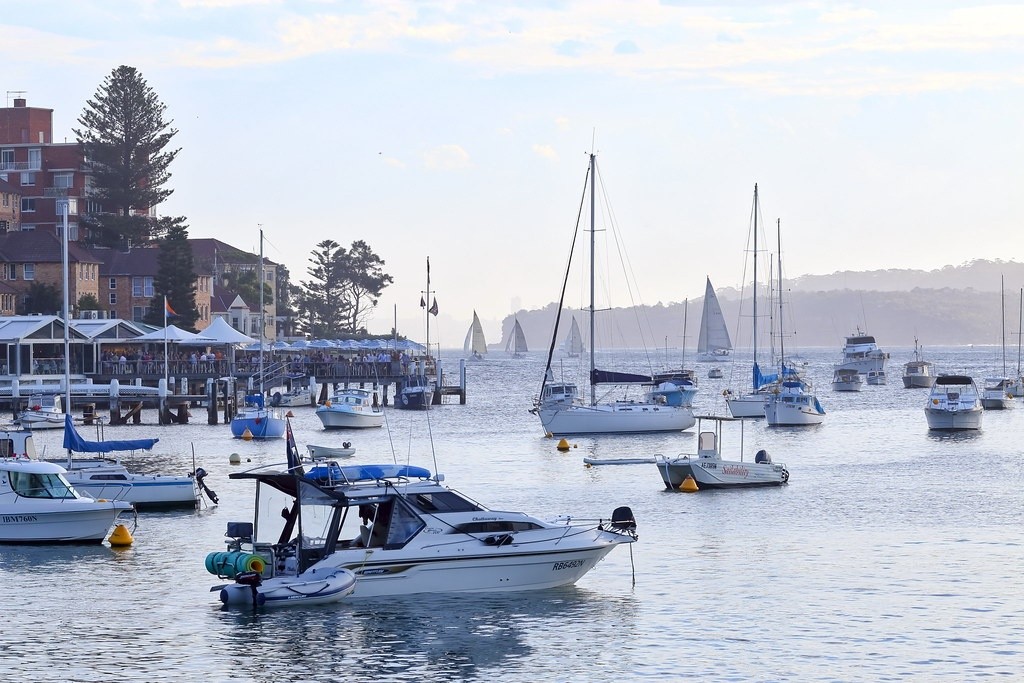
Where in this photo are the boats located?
[315,387,385,430]
[206,374,310,407]
[830,301,934,391]
[924,375,983,430]
[644,299,699,406]
[206,418,640,614]
[17,394,75,431]
[0,427,132,546]
[653,413,788,491]
[306,441,356,461]
[709,368,724,380]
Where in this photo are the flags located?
[166,301,182,320]
[420,297,426,307]
[429,297,438,316]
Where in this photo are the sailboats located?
[230,225,286,440]
[398,256,439,409]
[33,191,220,507]
[980,272,1024,409]
[697,270,733,362]
[462,309,488,362]
[564,313,586,358]
[504,312,530,358]
[527,151,696,435]
[723,183,828,426]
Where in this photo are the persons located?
[103,349,226,373]
[243,352,410,375]
[32,354,64,374]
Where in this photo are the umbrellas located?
[133,317,256,353]
[240,338,426,356]
